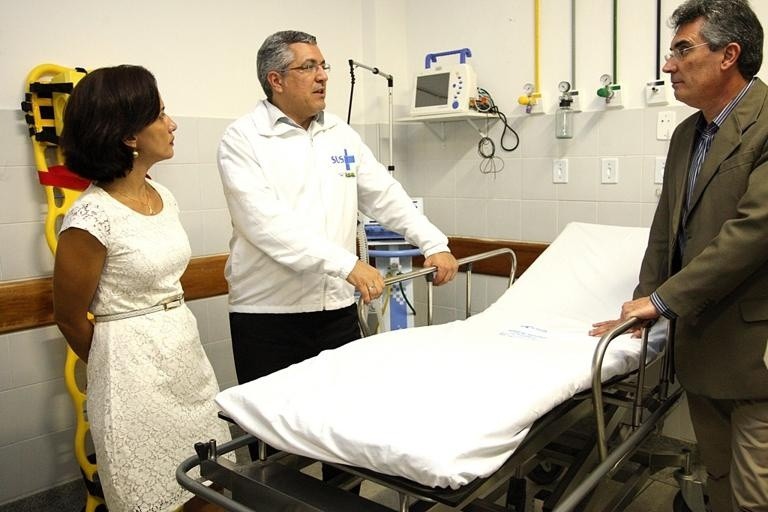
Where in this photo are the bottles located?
[555,101,574,139]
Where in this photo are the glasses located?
[664,41,712,62]
[280,63,331,73]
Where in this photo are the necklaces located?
[111,187,154,214]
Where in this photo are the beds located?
[206,221,680,506]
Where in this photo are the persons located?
[53,64,237,512]
[216,30,459,499]
[589,0,768,512]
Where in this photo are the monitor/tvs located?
[412,63,481,116]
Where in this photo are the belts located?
[94,295,184,322]
